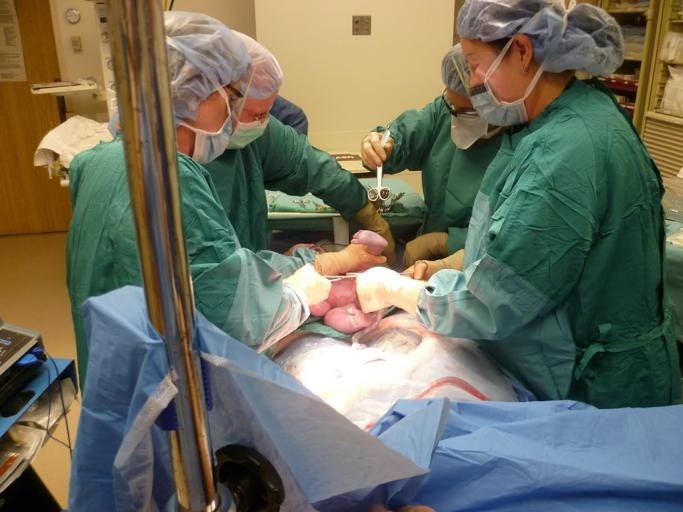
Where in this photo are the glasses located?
[441,88,480,119]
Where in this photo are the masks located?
[223,118,268,153]
[189,112,231,167]
[447,114,503,151]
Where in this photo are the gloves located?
[314,243,387,273]
[401,231,447,268]
[284,263,332,304]
[401,248,464,281]
[355,267,423,316]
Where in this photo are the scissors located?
[366,140,390,201]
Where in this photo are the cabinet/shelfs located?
[588,0,683,188]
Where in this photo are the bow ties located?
[470,86,529,127]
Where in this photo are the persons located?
[349,1,683,411]
[200,31,397,269]
[65,9,387,420]
[286,229,389,332]
[359,40,507,272]
